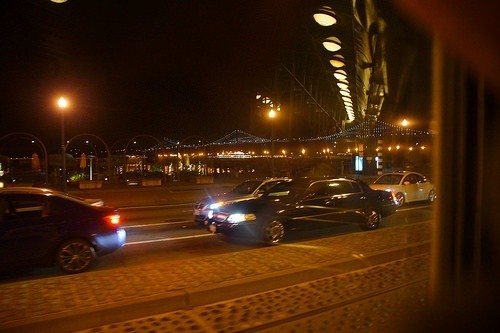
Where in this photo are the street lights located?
[401,119,409,161]
[56,96,67,193]
[268,110,277,176]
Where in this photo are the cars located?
[0,185,127,276]
[366,170,438,209]
[208,175,399,247]
[193,174,293,228]
